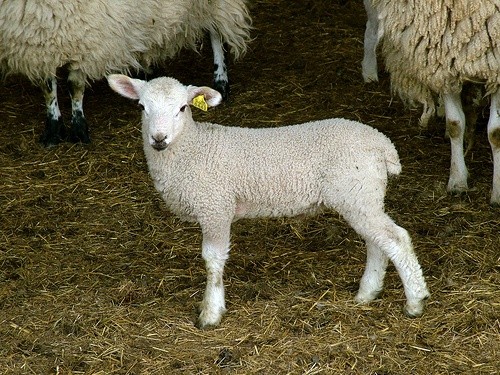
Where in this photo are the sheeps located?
[107,74,430,330]
[0,0,258,143]
[359,0,500,208]
